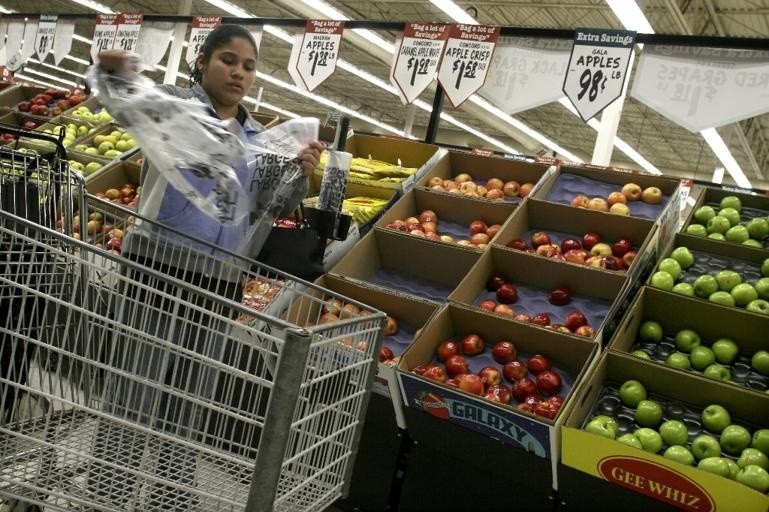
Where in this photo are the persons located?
[99,24,326,512]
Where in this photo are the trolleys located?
[0,119,399,511]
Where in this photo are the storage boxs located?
[247,111,768,512]
[0,81,147,288]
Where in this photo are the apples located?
[0,87,143,257]
[304,298,399,368]
[430,173,533,200]
[413,334,563,418]
[586,380,769,491]
[572,183,662,216]
[508,232,636,273]
[649,247,769,315]
[686,195,769,250]
[390,210,501,250]
[481,275,596,339]
[632,321,769,386]
